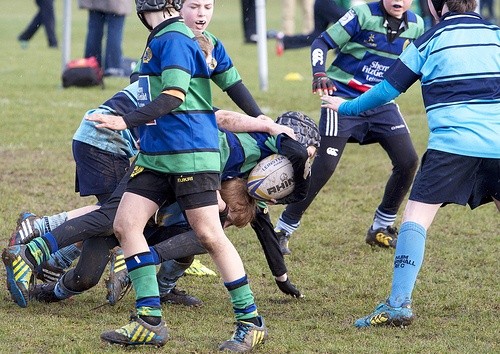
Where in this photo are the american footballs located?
[247,154,296,201]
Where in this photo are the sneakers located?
[106,247,133,307]
[353,302,412,328]
[25,284,60,302]
[219,317,267,354]
[2,245,38,308]
[182,257,217,276]
[100,315,168,347]
[365,226,399,249]
[8,212,41,248]
[161,287,203,308]
[41,262,64,285]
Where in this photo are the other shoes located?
[276,33,284,58]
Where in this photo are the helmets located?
[136,0,183,13]
[277,112,321,150]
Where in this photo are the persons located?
[273,0,425,256]
[320,0,500,328]
[1,0,496,308]
[85,0,271,353]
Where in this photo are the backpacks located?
[63,58,103,87]
